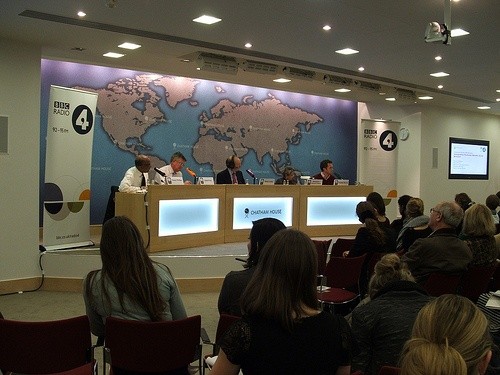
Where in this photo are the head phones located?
[228,155,235,168]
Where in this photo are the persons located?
[216,155,246,184]
[207,227,353,375]
[83,215,210,348]
[274,167,297,185]
[218,218,288,314]
[312,159,337,185]
[118,154,152,194]
[154,151,193,185]
[343,191,500,375]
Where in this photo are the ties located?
[140,174,146,186]
[232,172,238,185]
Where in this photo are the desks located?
[115,184,375,253]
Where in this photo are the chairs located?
[427,271,464,297]
[466,258,500,298]
[330,238,355,257]
[102,185,119,225]
[312,239,332,285]
[0,315,99,375]
[317,254,367,314]
[96,315,202,375]
[201,313,241,375]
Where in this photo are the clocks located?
[398,127,409,141]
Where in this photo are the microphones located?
[186,168,196,184]
[153,168,167,182]
[246,169,256,184]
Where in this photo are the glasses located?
[430,208,441,214]
